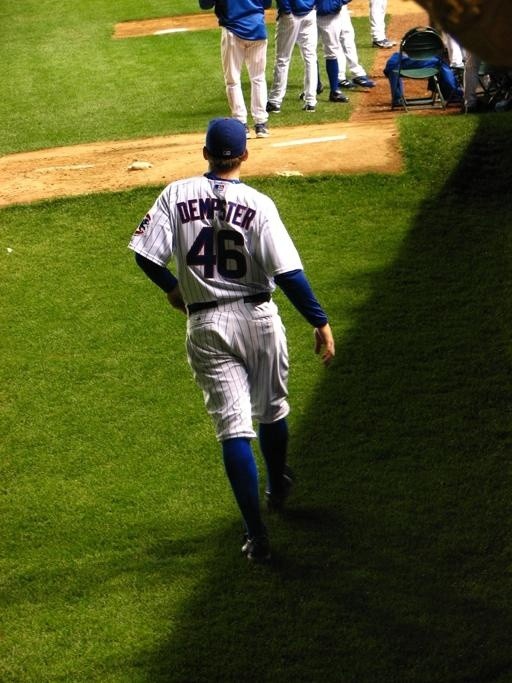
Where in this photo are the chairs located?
[386,25,447,113]
[445,53,495,116]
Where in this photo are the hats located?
[206,119,246,160]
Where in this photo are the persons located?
[336,0,379,90]
[267,0,321,114]
[198,0,274,139]
[127,118,336,563]
[460,50,492,113]
[298,0,351,103]
[369,0,397,49]
[443,31,469,76]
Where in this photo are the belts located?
[188,292,271,314]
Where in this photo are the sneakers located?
[329,76,374,103]
[373,39,397,49]
[243,123,268,139]
[267,102,281,113]
[266,465,295,513]
[240,530,271,562]
[302,105,315,113]
[300,83,323,99]
